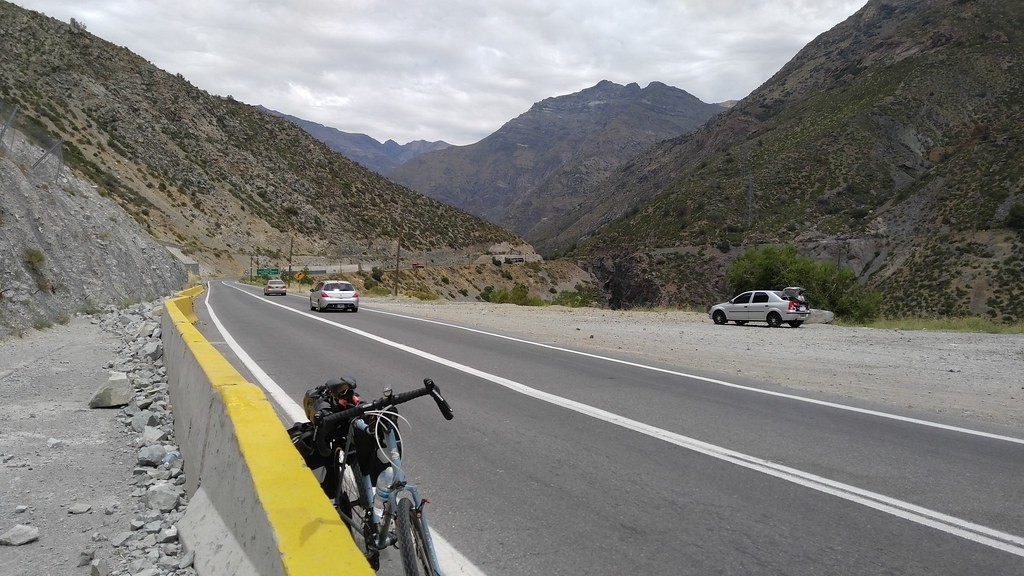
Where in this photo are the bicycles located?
[284,375,454,576]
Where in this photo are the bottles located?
[373,467,393,517]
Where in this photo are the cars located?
[708,287,812,328]
[310,279,360,312]
[263,279,287,296]
